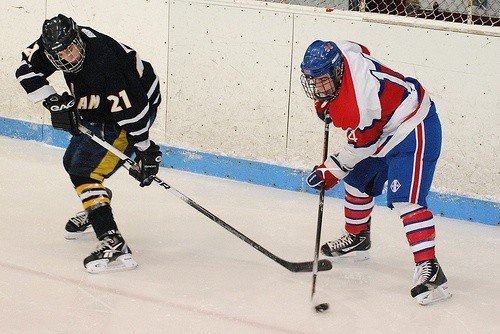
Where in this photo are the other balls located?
[314,303,330,313]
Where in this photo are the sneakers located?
[84,229,138,274]
[63,210,93,239]
[320,228,372,263]
[411,257,453,304]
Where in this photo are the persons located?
[299,39,452,306]
[15,13,162,271]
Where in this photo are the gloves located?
[42,90,84,137]
[305,153,354,191]
[129,139,162,187]
[314,97,333,124]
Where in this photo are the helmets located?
[41,13,85,75]
[300,40,345,103]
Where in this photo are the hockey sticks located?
[311,112,333,313]
[77,122,333,273]
